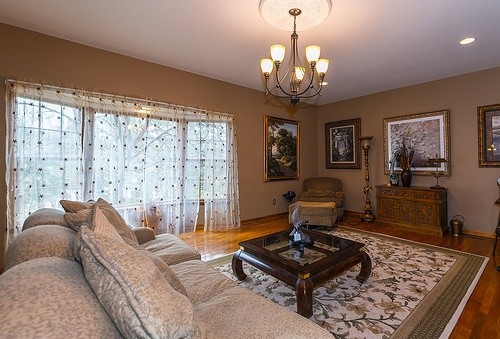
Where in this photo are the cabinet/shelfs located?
[375,185,447,237]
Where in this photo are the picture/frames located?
[383,109,451,177]
[476,104,500,168]
[324,117,362,169]
[264,115,300,182]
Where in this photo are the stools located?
[289,201,337,229]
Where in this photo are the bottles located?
[294,231,301,242]
[390,170,399,187]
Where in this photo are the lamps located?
[358,135,373,224]
[259,8,331,106]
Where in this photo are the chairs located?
[299,177,344,221]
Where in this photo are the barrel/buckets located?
[450,215,464,236]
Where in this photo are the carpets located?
[206,223,491,339]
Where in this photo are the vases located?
[400,170,412,187]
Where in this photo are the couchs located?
[0,208,335,339]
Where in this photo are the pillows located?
[85,204,126,243]
[60,198,95,213]
[136,248,187,296]
[73,227,199,339]
[64,198,139,243]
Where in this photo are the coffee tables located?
[232,226,372,319]
